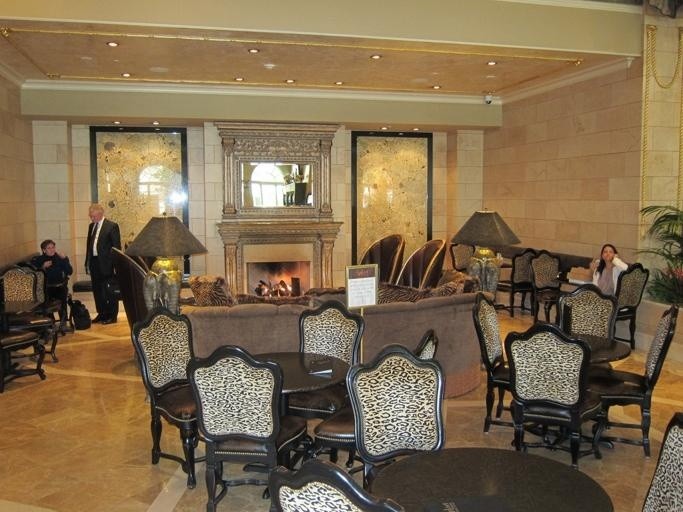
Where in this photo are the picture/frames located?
[90,126,191,279]
[352,129,433,262]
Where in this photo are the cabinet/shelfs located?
[283,181,306,206]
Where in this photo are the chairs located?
[559,282,620,430]
[504,322,604,468]
[110,247,151,332]
[347,347,446,490]
[315,330,438,466]
[641,411,683,512]
[0,252,92,394]
[187,345,305,511]
[472,293,547,440]
[131,307,201,488]
[450,243,649,350]
[122,242,149,273]
[358,234,406,290]
[266,459,406,511]
[392,237,447,290]
[589,307,678,459]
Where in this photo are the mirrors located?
[232,155,320,217]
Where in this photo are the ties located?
[90,223,98,256]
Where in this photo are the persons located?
[84,204,120,324]
[33,239,72,335]
[592,244,628,296]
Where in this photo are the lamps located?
[123,215,207,312]
[453,209,521,302]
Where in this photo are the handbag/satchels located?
[72,300,91,330]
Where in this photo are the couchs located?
[178,275,487,402]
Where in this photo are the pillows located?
[187,277,465,307]
